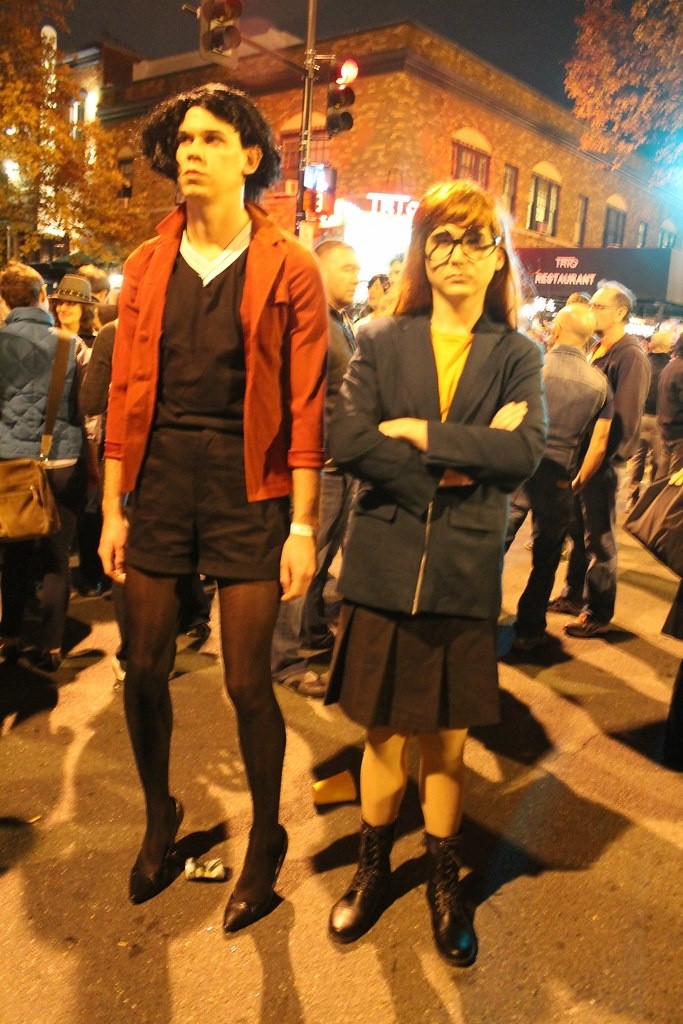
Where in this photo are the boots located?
[422,835,480,965]
[324,816,398,940]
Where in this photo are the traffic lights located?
[327,52,358,135]
[200,0,243,71]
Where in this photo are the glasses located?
[423,229,503,261]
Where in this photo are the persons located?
[324,177,551,968]
[0,242,683,768]
[96,86,332,932]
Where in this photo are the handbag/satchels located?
[623,476,683,577]
[1,457,61,540]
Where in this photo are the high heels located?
[222,825,288,935]
[128,798,185,904]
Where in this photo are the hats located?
[47,275,94,305]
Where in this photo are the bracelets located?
[288,524,315,538]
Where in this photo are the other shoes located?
[1,641,24,662]
[563,610,611,636]
[548,590,583,612]
[515,632,551,649]
[281,672,327,699]
[29,648,64,671]
[85,577,107,598]
